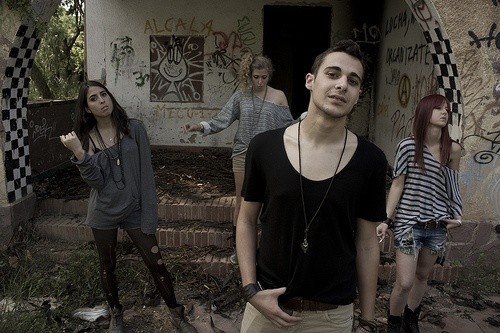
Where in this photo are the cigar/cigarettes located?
[379,238,383,243]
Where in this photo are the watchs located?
[240,280,265,303]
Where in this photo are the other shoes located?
[405,303,423,333]
[168,305,198,333]
[386,310,404,333]
[106,306,126,333]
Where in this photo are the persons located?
[183,47,294,265]
[235,37,388,333]
[377,95,463,333]
[59,81,198,333]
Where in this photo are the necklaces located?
[298,119,348,253]
[251,83,267,128]
[94,121,125,185]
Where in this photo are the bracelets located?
[455,218,462,226]
[358,315,376,333]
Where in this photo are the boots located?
[229,225,239,265]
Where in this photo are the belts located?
[416,222,447,230]
[279,296,338,312]
